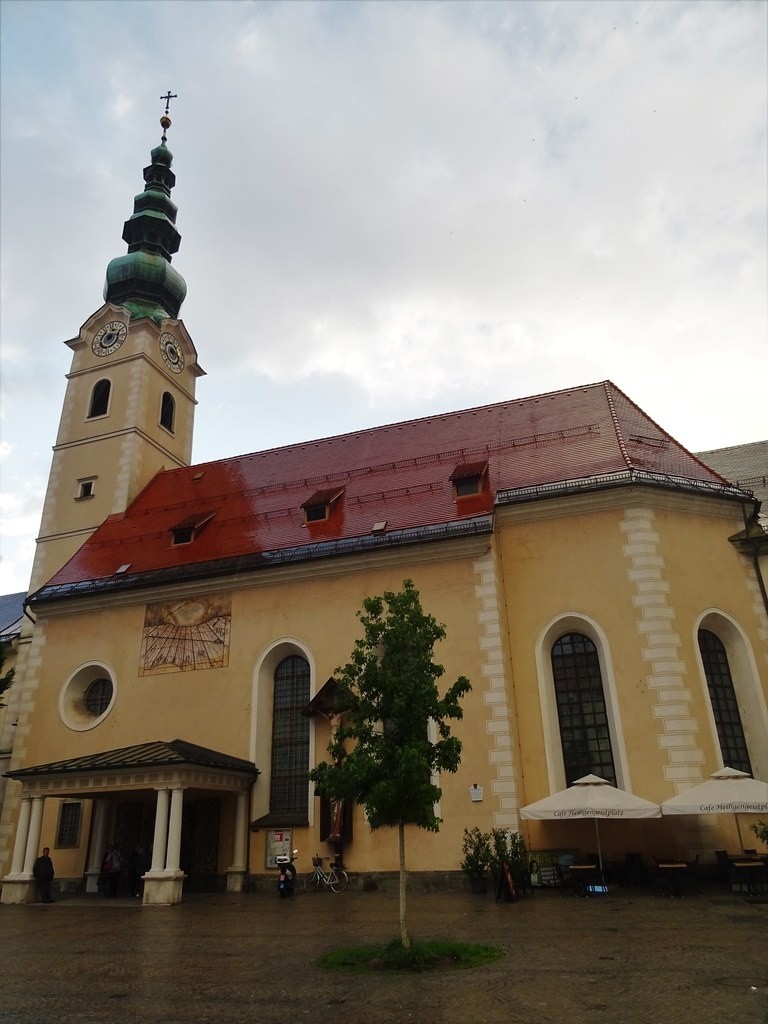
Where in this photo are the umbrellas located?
[659,766,768,857]
[519,773,663,884]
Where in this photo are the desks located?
[569,864,597,897]
[732,861,765,896]
[657,863,687,898]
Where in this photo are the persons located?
[529,860,538,884]
[33,847,57,903]
[97,842,146,898]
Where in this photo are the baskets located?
[313,858,323,866]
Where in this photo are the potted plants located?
[461,827,493,894]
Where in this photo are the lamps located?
[372,521,387,535]
[115,564,133,576]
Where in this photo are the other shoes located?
[41,898,55,904]
[102,887,143,899]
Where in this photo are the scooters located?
[275,849,299,900]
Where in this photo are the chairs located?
[650,855,699,898]
[551,853,607,899]
[715,850,764,892]
[490,860,538,898]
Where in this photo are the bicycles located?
[303,852,349,893]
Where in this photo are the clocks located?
[159,332,185,373]
[91,321,127,357]
